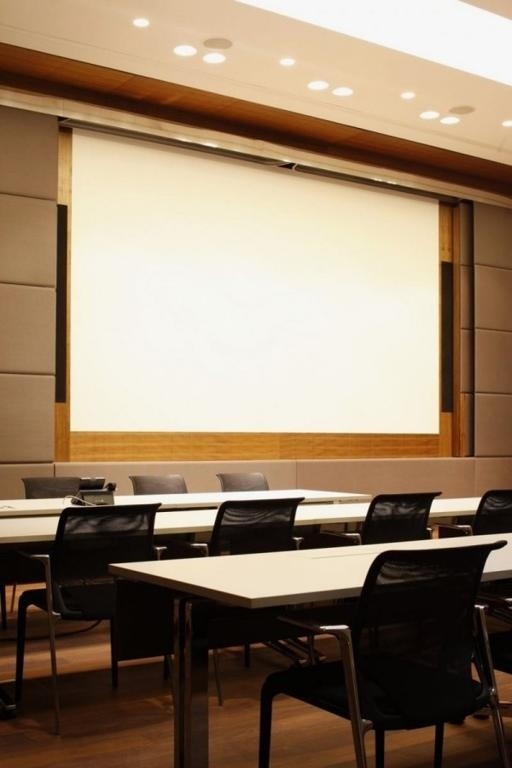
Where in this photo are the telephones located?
[71,477,117,506]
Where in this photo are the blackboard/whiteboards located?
[52,205,455,415]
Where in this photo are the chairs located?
[256,539,508,767]
[0,471,512,736]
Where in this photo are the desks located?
[109,532,512,768]
